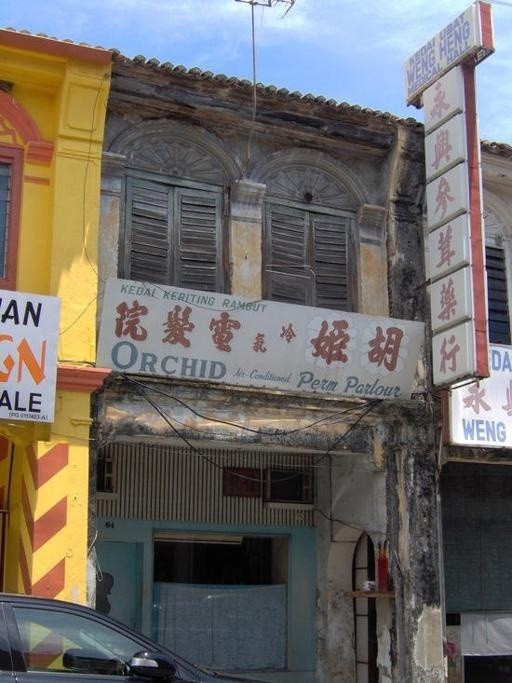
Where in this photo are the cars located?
[1,591,211,682]
